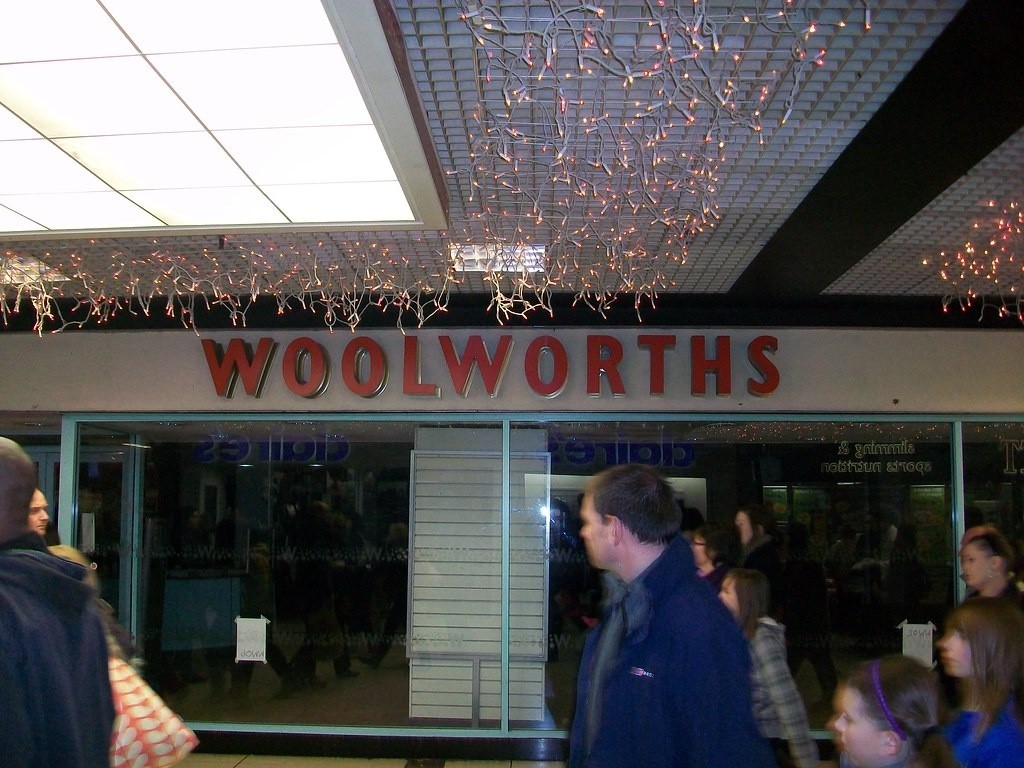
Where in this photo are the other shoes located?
[216,687,248,705]
[335,667,359,680]
[272,671,303,702]
[810,684,836,712]
[301,674,326,688]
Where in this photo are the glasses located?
[689,537,707,547]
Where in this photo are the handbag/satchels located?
[103,656,200,768]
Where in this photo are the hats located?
[249,542,270,567]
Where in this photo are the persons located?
[779,505,928,707]
[568,568,629,768]
[164,493,408,688]
[0,436,135,768]
[937,596,1024,768]
[579,462,771,768]
[834,655,956,768]
[960,526,1024,604]
[686,503,822,768]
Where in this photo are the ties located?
[358,656,380,671]
[587,585,629,758]
